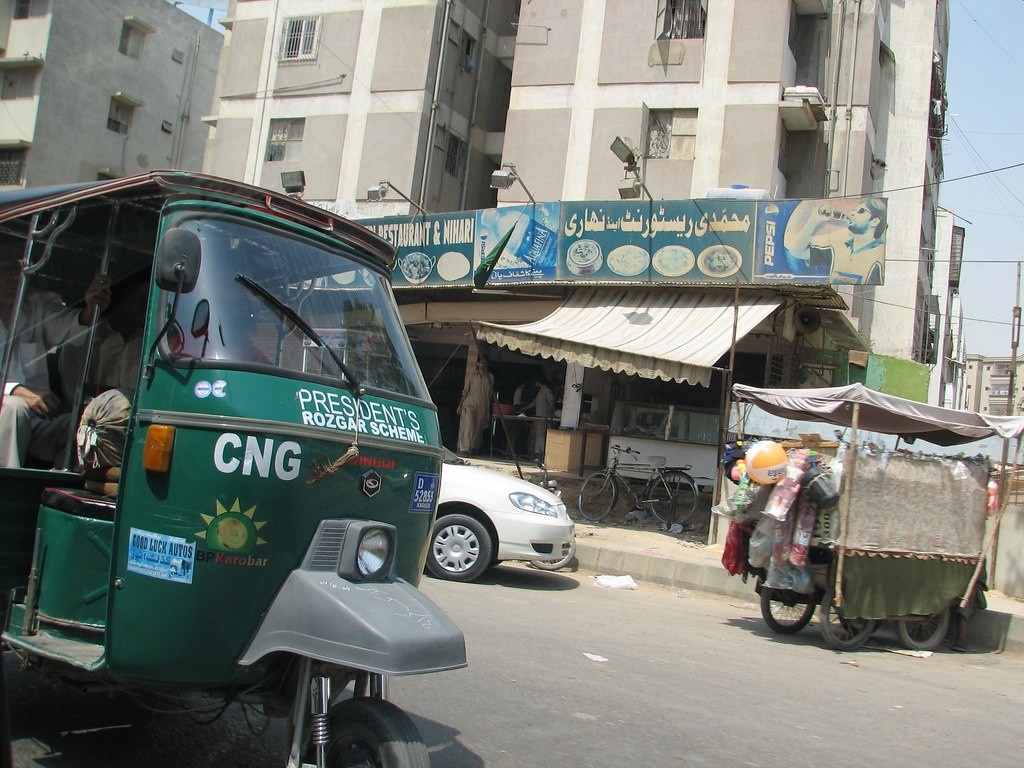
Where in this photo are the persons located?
[509,378,554,463]
[457,358,494,458]
[988,482,997,510]
[168,273,272,365]
[0,244,112,467]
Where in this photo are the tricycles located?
[715,382,1024,651]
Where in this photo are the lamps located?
[366,180,429,215]
[490,163,537,205]
[280,170,306,199]
[610,136,653,201]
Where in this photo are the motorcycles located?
[0,168,472,768]
[526,456,579,572]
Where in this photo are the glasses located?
[853,207,872,215]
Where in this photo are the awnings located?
[473,288,786,388]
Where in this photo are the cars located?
[423,445,574,582]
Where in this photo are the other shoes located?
[458,451,472,458]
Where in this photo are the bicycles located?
[577,442,700,530]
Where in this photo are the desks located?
[491,415,560,462]
